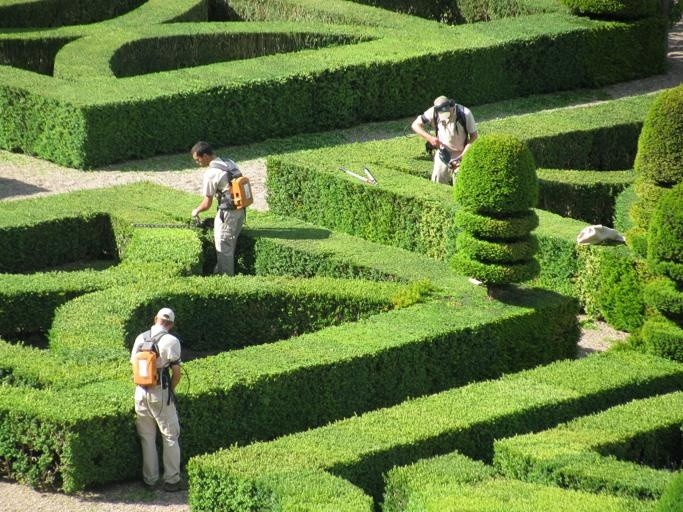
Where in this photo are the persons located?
[191,141,246,275]
[131,307,189,492]
[411,95,477,187]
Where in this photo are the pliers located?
[339,167,377,184]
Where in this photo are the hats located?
[158,308,175,322]
[434,96,450,120]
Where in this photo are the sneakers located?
[143,476,189,491]
